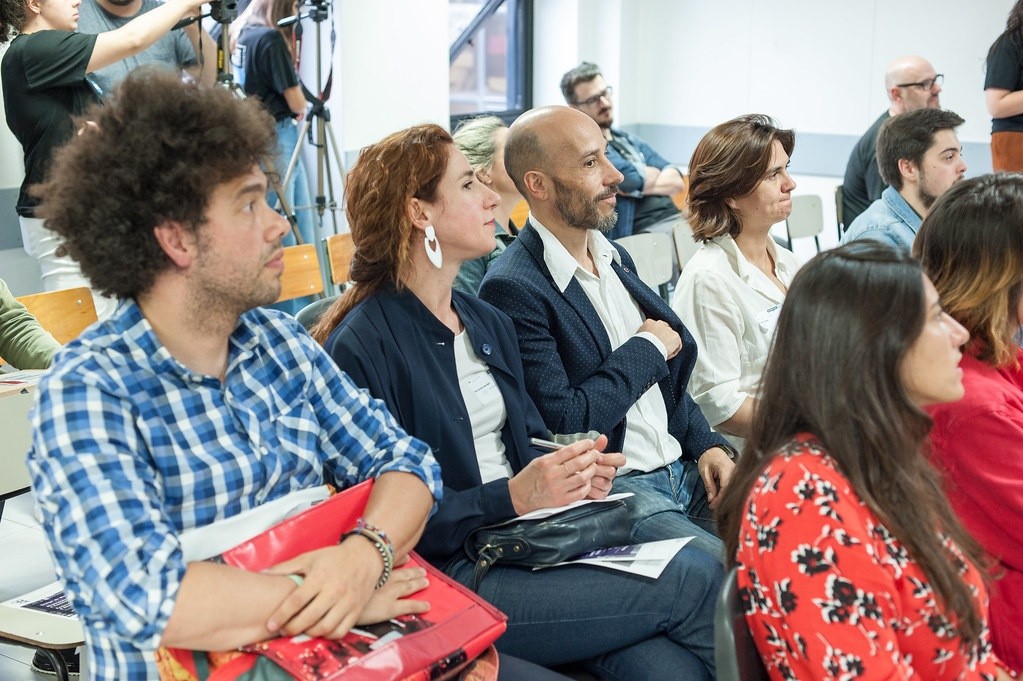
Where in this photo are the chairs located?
[0,175,844,681]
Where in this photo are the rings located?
[564,462,571,475]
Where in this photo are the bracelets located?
[287,575,303,586]
[339,519,395,590]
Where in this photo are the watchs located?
[714,444,735,460]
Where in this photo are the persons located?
[453,62,809,566]
[0,0,323,322]
[28,63,573,681]
[711,57,1023,679]
[0,278,63,369]
[984,1,1023,181]
[303,123,725,680]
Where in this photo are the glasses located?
[896,74,944,90]
[576,86,613,108]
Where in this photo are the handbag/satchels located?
[464,499,630,594]
[154,477,509,681]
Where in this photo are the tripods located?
[172,0,350,299]
